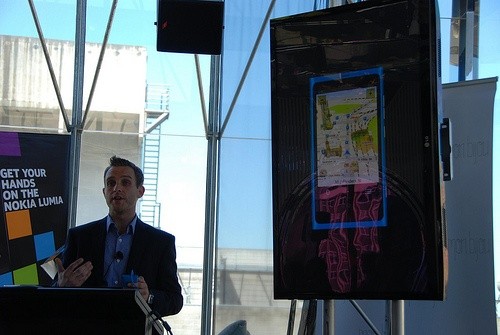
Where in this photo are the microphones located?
[98,251,122,288]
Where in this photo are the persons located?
[51,154,183,317]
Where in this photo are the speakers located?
[154,0,225,55]
